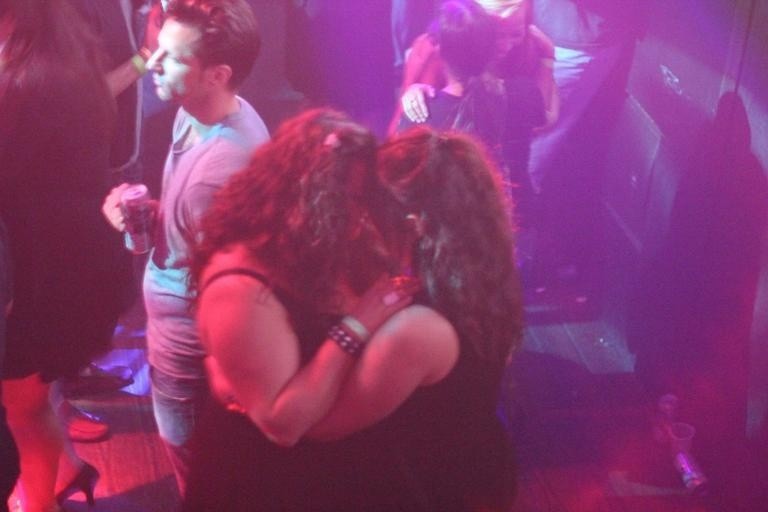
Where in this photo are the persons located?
[192,107,421,509]
[396,1,654,296]
[204,127,524,511]
[100,0,271,512]
[3,1,98,510]
[49,0,165,441]
[670,90,768,433]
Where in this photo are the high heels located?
[56,457,98,506]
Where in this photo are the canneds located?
[120,184,152,255]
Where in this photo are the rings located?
[382,293,401,306]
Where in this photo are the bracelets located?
[339,315,371,343]
[325,325,361,359]
[130,54,146,75]
[139,47,152,57]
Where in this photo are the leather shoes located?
[61,359,133,443]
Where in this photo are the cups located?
[669,422,696,455]
